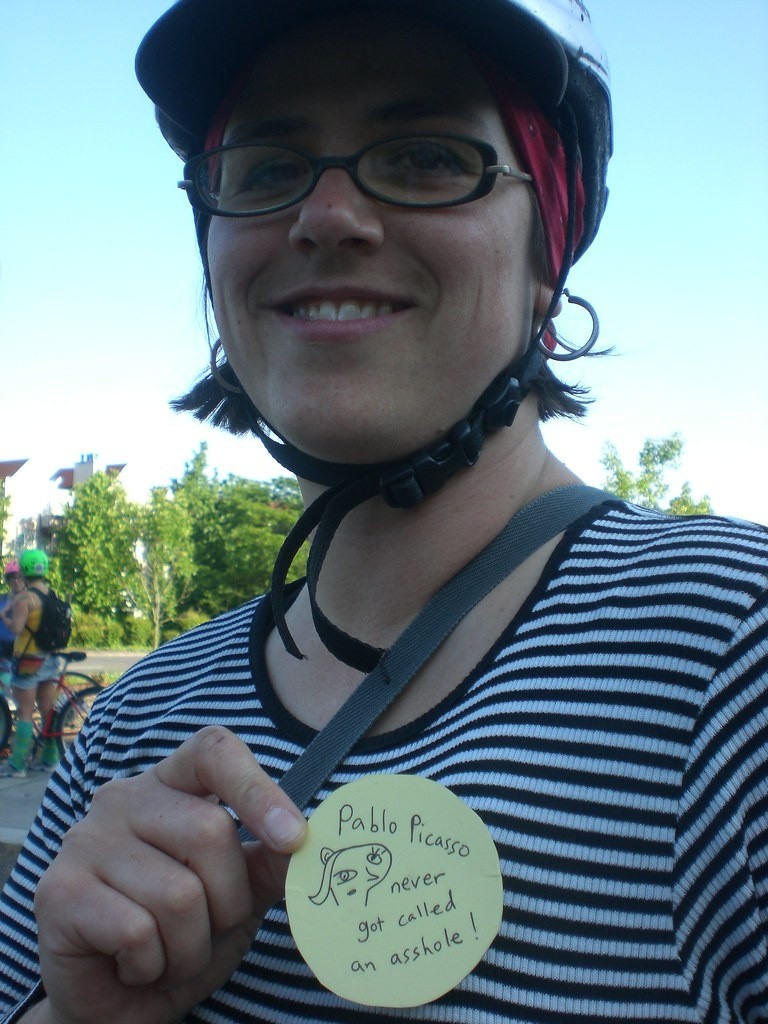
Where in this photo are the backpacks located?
[23,587,72,652]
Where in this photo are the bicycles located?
[0,651,108,757]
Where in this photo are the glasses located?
[178,131,534,218]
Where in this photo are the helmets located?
[135,0,614,267]
[3,560,19,576]
[19,549,48,578]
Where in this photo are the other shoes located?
[0,764,25,778]
[29,761,54,771]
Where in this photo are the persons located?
[0,0,768,1024]
[0,560,25,660]
[0,549,61,777]
